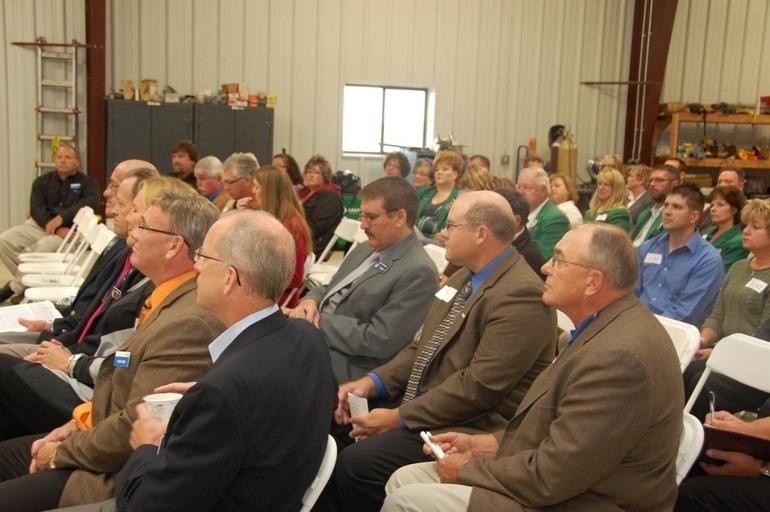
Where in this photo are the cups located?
[142,391,185,425]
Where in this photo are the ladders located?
[35,37,80,177]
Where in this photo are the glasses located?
[224,176,246,184]
[446,219,481,232]
[137,216,192,250]
[385,161,400,168]
[358,209,400,221]
[413,168,431,178]
[193,247,243,287]
[649,177,674,184]
[550,254,598,272]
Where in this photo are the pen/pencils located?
[708,390,715,426]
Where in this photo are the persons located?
[629,185,727,328]
[2,187,220,505]
[383,152,750,270]
[676,409,770,511]
[379,221,685,510]
[1,141,102,308]
[332,186,558,511]
[172,141,341,310]
[685,197,770,405]
[0,160,198,439]
[282,175,439,383]
[49,210,337,511]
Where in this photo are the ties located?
[319,253,382,313]
[401,279,473,404]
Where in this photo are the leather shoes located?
[11,290,31,303]
[0,280,14,304]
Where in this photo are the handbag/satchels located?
[331,172,362,194]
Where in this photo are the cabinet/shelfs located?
[102,96,274,198]
[652,102,769,197]
